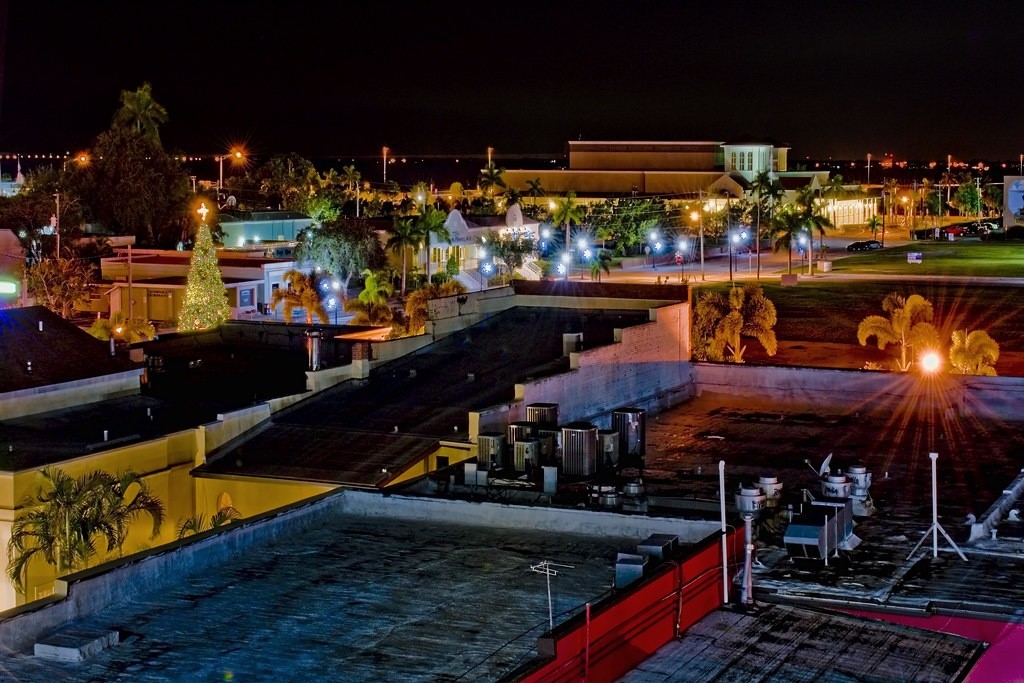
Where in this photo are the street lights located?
[331,279,342,324]
[732,233,741,271]
[477,249,488,291]
[678,239,688,281]
[61,153,88,174]
[649,229,659,268]
[579,237,587,277]
[218,150,244,190]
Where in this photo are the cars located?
[910,221,1000,238]
[845,239,882,252]
